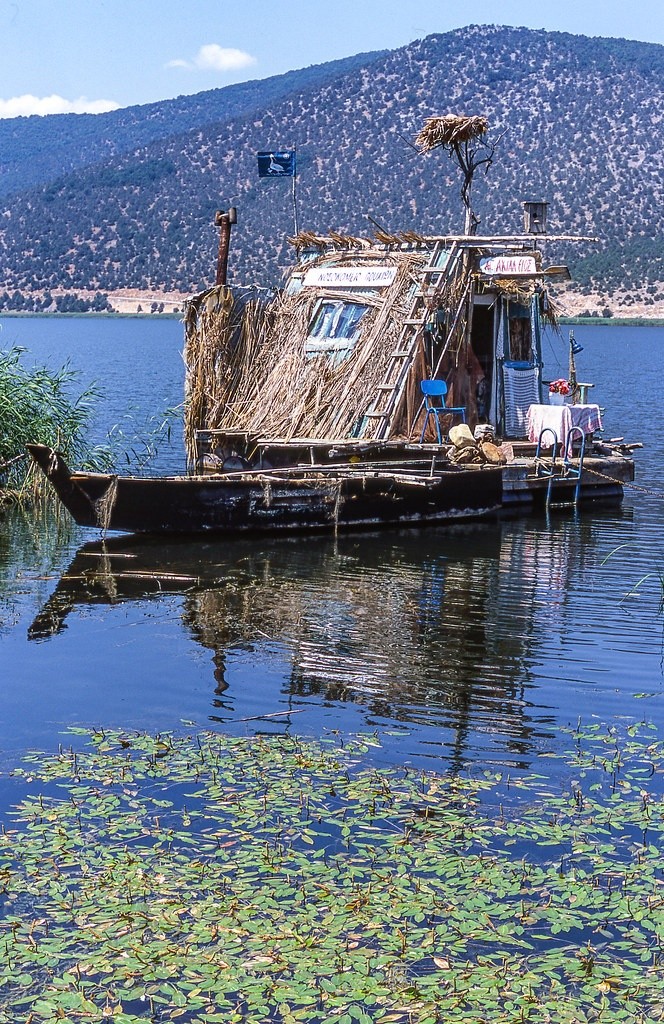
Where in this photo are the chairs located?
[419,379,467,446]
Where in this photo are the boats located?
[28,203,644,536]
[29,505,639,720]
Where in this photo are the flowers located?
[549,379,570,394]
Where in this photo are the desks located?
[526,404,603,459]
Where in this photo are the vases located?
[552,392,564,406]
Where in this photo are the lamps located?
[570,338,584,354]
